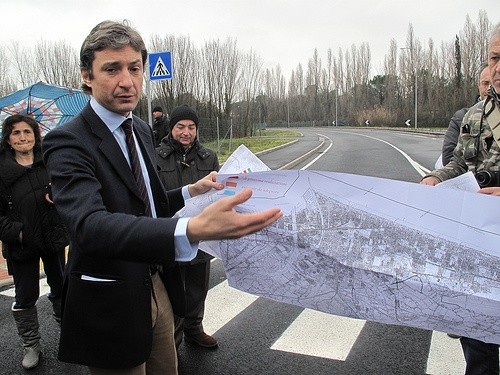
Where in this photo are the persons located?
[418,21,500,375]
[0,113,67,370]
[41,20,283,375]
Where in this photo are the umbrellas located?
[1,80,91,139]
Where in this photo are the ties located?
[121,118,153,218]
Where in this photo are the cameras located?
[473,169,500,189]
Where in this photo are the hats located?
[153,106,163,112]
[170,104,199,131]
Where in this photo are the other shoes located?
[184,332,219,346]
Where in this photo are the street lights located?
[400,47,418,130]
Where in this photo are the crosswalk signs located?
[149,51,173,81]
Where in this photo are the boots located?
[11,302,42,369]
[47,293,61,328]
